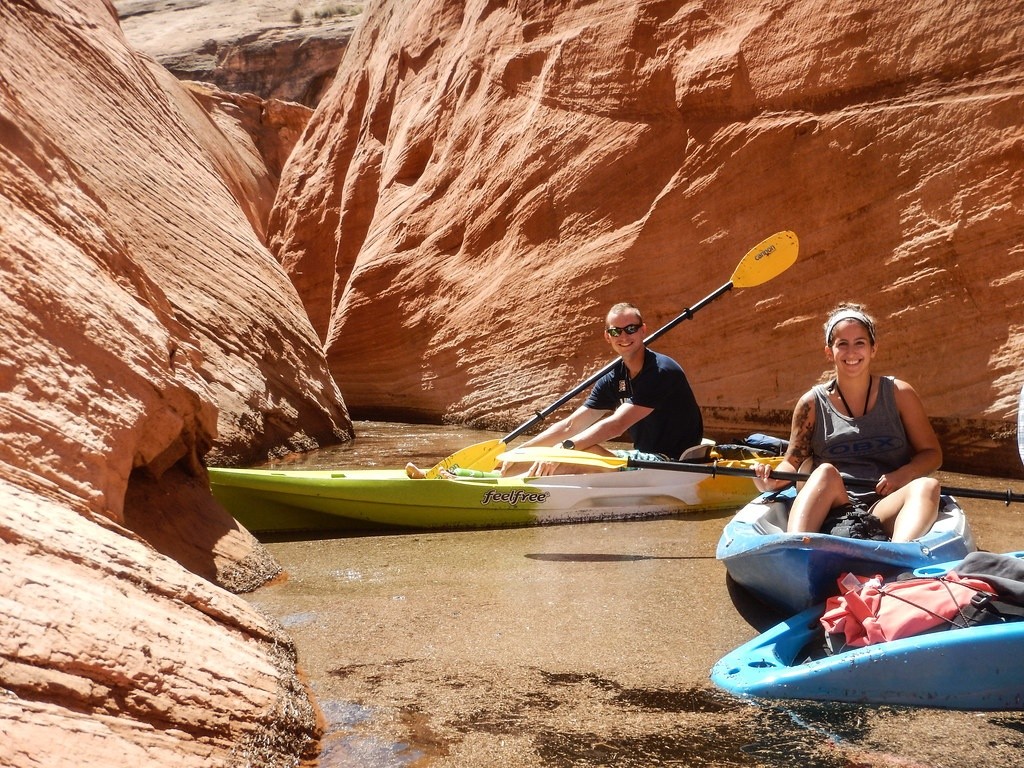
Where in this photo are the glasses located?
[607,324,643,337]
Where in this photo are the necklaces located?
[835,374,872,419]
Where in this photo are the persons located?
[405,302,704,479]
[750,302,942,543]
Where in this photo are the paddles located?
[422,231,801,480]
[495,449,1024,510]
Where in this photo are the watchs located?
[562,440,576,450]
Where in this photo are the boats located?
[714,473,977,612]
[708,550,1024,711]
[203,439,797,528]
